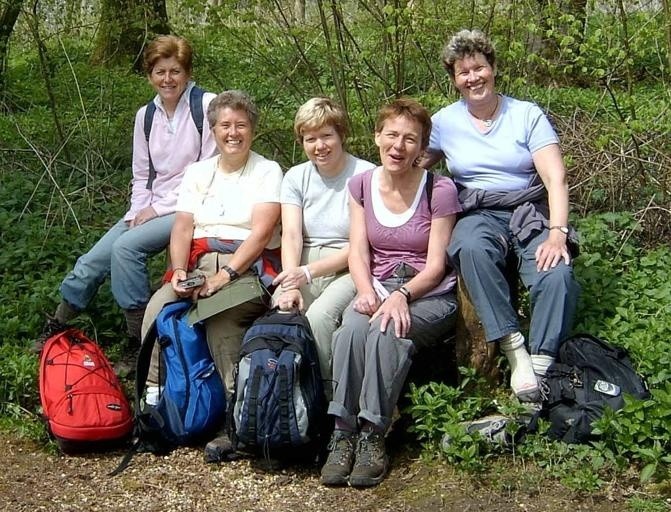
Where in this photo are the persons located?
[142,90,285,461]
[417,30,574,413]
[273,97,377,404]
[30,35,218,377]
[321,99,463,488]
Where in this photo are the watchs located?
[394,287,411,300]
[550,226,569,234]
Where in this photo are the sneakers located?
[440,415,525,458]
[28,313,76,354]
[508,373,550,429]
[206,428,241,460]
[113,329,141,380]
[321,423,392,487]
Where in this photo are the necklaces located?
[467,95,498,127]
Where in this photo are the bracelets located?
[221,266,239,281]
[173,268,187,272]
[301,266,312,284]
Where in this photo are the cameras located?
[178,275,206,289]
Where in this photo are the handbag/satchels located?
[540,332,650,449]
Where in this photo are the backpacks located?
[39,329,133,454]
[232,304,326,471]
[133,297,226,454]
